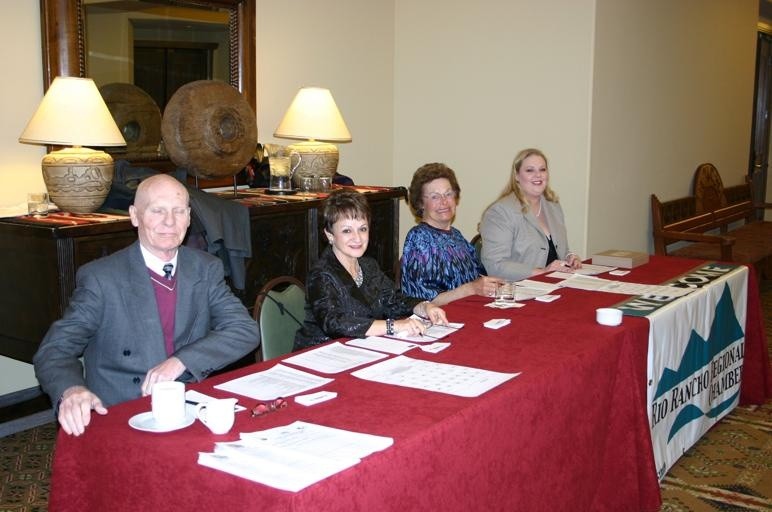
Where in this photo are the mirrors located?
[39,1,259,192]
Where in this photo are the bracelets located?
[564,253,578,258]
[385,317,395,336]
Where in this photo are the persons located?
[402,162,513,307]
[478,148,584,281]
[32,173,262,438]
[290,186,449,353]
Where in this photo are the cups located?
[318,174,333,193]
[150,380,187,421]
[596,307,623,327]
[26,192,50,218]
[299,173,316,194]
[494,280,516,305]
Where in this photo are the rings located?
[488,292,493,296]
[488,288,495,291]
[414,326,421,329]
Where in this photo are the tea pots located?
[196,398,238,436]
[262,142,302,191]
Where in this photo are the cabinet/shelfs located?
[1,208,139,367]
[195,177,406,313]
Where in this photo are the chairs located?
[469,234,483,266]
[248,275,308,365]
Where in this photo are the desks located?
[37,247,772,512]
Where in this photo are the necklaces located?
[533,198,543,219]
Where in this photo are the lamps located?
[16,74,131,215]
[270,87,354,189]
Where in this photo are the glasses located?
[252,397,287,417]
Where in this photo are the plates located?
[128,411,196,433]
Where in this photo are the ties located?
[163,264,172,280]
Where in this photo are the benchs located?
[646,160,770,296]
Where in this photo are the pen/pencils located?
[185,400,206,409]
[479,273,504,285]
